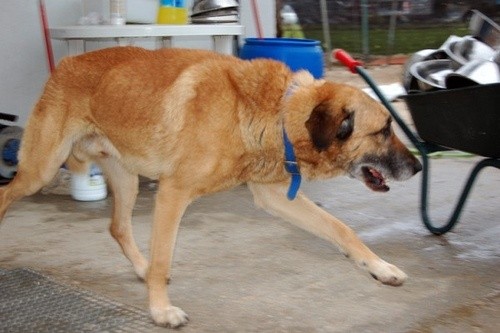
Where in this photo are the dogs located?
[1,46,423,328]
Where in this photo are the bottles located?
[71,164,107,201]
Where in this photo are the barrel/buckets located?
[240,38,323,78]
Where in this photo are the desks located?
[48,23,244,57]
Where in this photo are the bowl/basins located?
[409,59,463,91]
[445,37,497,65]
[189,0,240,23]
[401,49,443,95]
[468,9,500,47]
[445,60,500,89]
